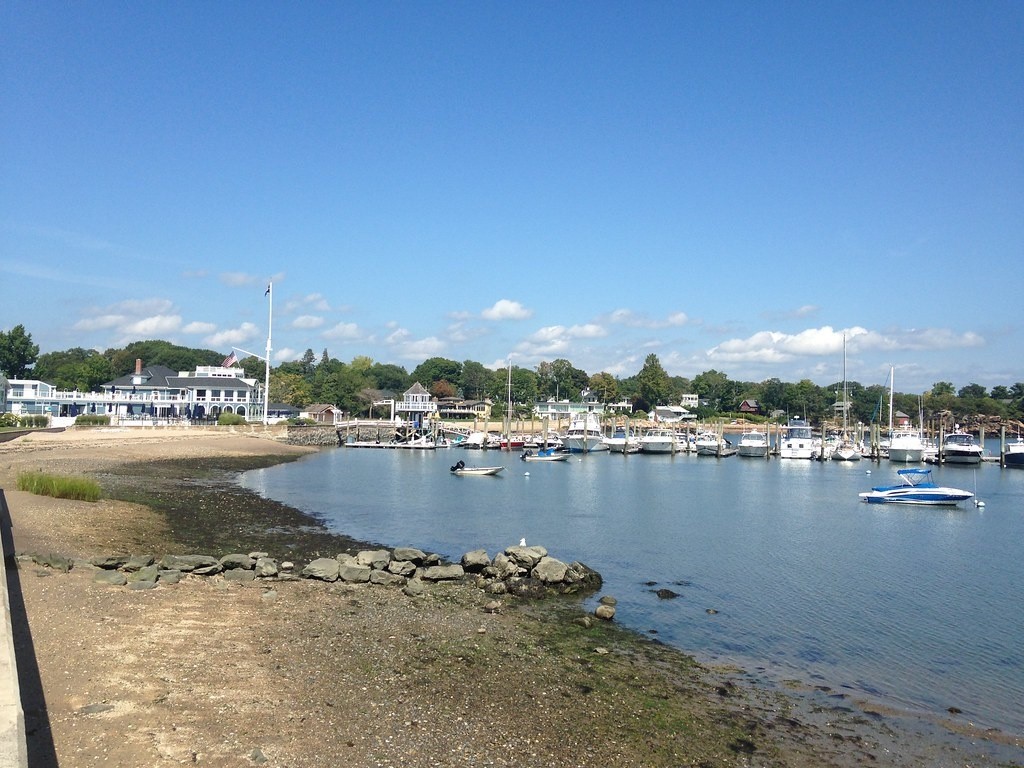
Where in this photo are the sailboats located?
[832,333,864,460]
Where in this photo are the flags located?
[221,351,237,368]
[264,284,270,297]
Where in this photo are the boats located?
[736,430,769,457]
[603,416,646,454]
[342,360,563,449]
[521,448,574,463]
[448,460,504,476]
[559,408,608,452]
[858,469,974,506]
[938,434,982,465]
[641,423,699,453]
[696,417,739,455]
[887,430,924,462]
[1004,440,1024,465]
[781,420,830,461]
